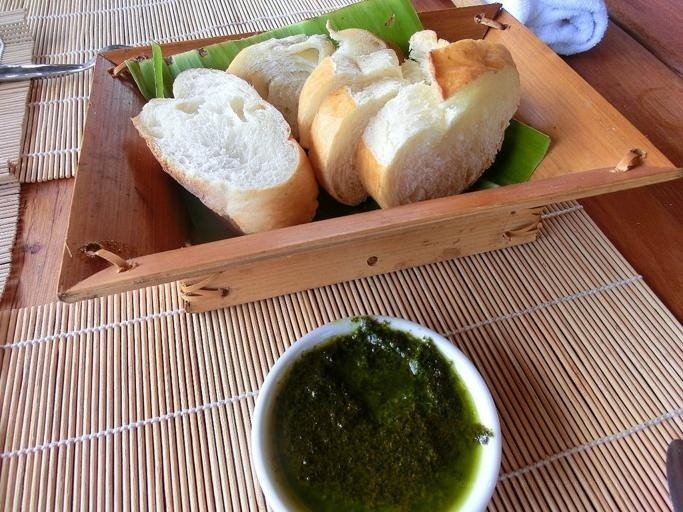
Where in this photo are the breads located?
[131,19,522,234]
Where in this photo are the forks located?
[1,41,163,86]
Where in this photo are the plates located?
[251,313,501,512]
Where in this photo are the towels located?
[486,0,609,56]
